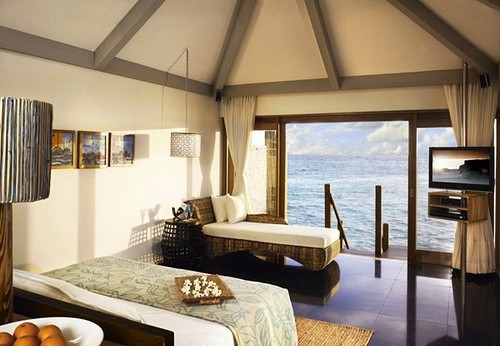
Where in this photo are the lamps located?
[170,48,202,157]
[0,97,53,325]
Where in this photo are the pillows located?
[210,195,228,223]
[226,193,247,224]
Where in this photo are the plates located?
[0,317,104,346]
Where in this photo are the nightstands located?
[161,218,205,271]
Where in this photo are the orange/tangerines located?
[0,322,68,346]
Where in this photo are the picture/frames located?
[77,131,108,168]
[108,133,136,167]
[51,129,77,169]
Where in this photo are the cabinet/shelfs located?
[428,192,489,223]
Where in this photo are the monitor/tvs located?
[429,146,494,192]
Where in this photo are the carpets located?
[294,316,375,346]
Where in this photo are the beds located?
[183,196,341,271]
[13,256,299,346]
